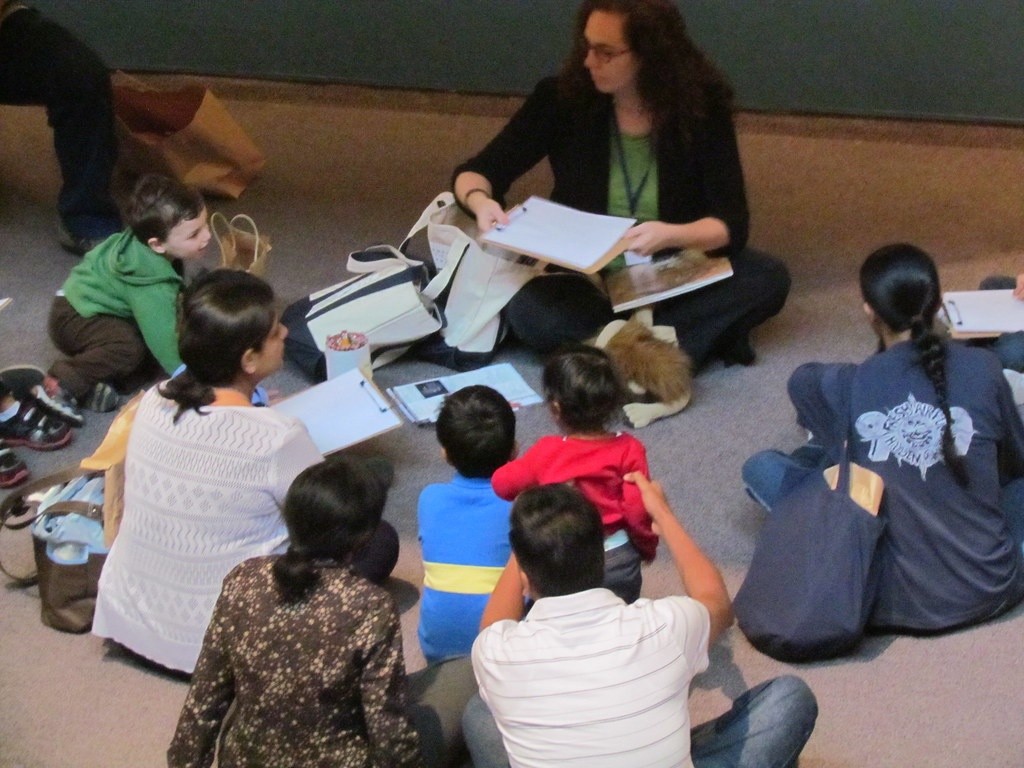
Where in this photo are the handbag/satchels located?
[0,466,109,633]
[112,69,267,201]
[732,362,889,661]
[398,190,548,372]
[211,212,272,280]
[281,245,443,383]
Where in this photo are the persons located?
[450,0,789,405]
[491,343,660,605]
[31,176,212,423]
[0,2,121,250]
[0,380,72,486]
[462,471,819,768]
[967,271,1024,423]
[742,243,1024,631]
[92,267,325,673]
[417,384,531,665]
[167,457,478,768]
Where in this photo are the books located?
[607,248,734,313]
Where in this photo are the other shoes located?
[58,216,118,250]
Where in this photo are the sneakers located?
[78,381,119,412]
[0,443,27,487]
[0,397,73,448]
[30,376,84,426]
[0,365,44,401]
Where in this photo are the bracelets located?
[465,188,491,210]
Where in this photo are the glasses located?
[575,36,632,63]
